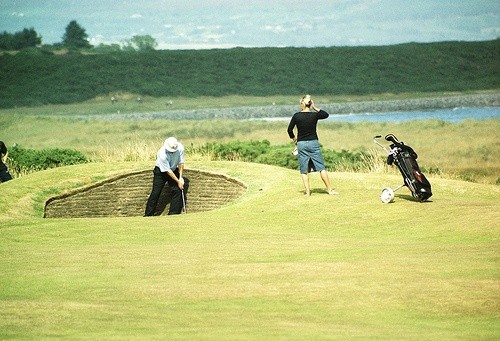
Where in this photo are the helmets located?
[380,188,394,203]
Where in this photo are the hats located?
[164,137,179,152]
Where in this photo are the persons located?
[287,95,339,195]
[0,140,12,184]
[143,136,185,217]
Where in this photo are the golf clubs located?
[372,135,401,157]
[182,189,186,214]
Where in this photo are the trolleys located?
[373,136,424,204]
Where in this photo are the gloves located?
[179,178,185,185]
